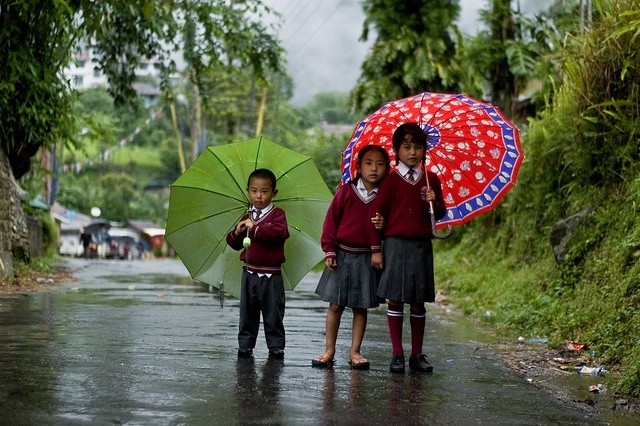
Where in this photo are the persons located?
[368,123,447,372]
[311,145,392,367]
[226,168,286,357]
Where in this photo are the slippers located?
[348,356,370,367]
[312,355,337,365]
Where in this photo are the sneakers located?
[238,347,253,355]
[268,349,284,357]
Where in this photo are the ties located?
[255,211,262,220]
[407,169,415,181]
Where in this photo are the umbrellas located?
[340,91,523,240]
[164,136,334,300]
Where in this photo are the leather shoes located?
[390,353,405,372]
[409,354,433,371]
